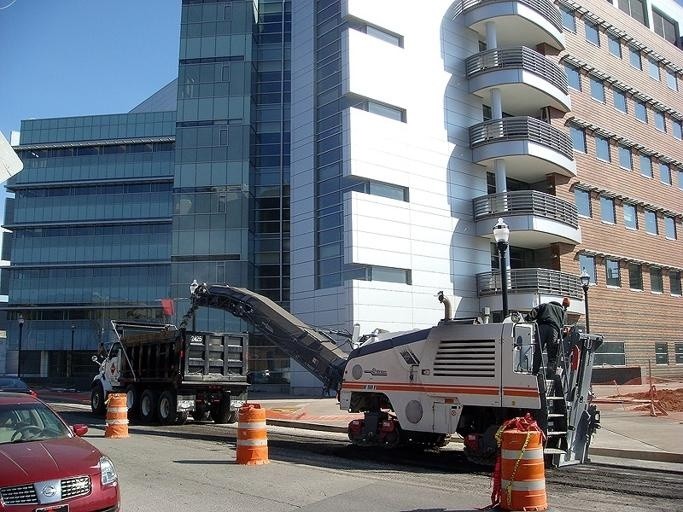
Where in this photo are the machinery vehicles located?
[185,278,605,472]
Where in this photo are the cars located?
[0,372,39,405]
[1,390,123,512]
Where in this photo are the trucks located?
[85,310,248,431]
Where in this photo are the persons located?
[525,302,564,380]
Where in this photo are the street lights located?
[578,261,595,334]
[492,215,514,318]
[15,313,25,379]
[68,322,76,383]
